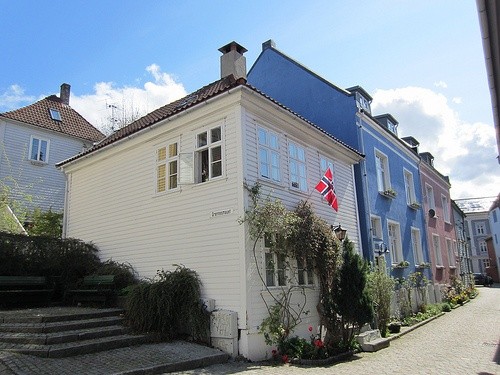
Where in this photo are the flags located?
[315,166,338,212]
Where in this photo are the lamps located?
[380,242,390,252]
[331,224,347,242]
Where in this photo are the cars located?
[472,273,493,288]
